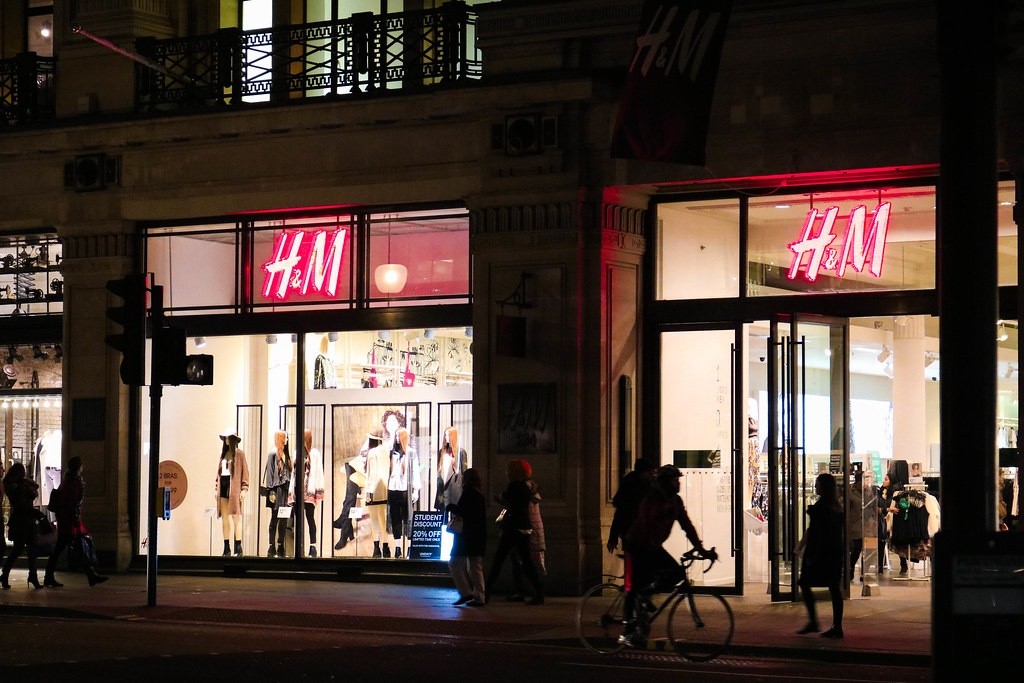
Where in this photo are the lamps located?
[424,329,435,339]
[378,330,389,339]
[877,346,890,362]
[1002,363,1015,379]
[328,333,338,342]
[465,327,473,336]
[375,263,408,294]
[998,325,1009,340]
[193,337,205,347]
[924,353,936,368]
[291,335,298,343]
[266,336,277,344]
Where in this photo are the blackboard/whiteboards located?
[410,511,443,560]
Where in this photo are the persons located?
[467,458,546,606]
[267,430,292,558]
[881,474,908,574]
[794,473,847,640]
[366,427,392,557]
[215,433,249,556]
[0,463,43,589]
[34,431,61,525]
[848,473,881,582]
[331,410,418,550]
[605,458,654,623]
[389,430,422,558]
[314,353,337,389]
[288,430,324,557]
[620,462,718,650]
[434,428,467,526]
[446,467,487,605]
[44,457,110,586]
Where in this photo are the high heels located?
[0,573,11,588]
[27,571,43,589]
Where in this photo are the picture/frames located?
[0,446,24,471]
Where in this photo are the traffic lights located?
[105,273,146,387]
[181,354,214,388]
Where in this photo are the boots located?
[309,545,317,558]
[367,541,411,559]
[44,566,64,587]
[334,461,362,549]
[86,566,108,587]
[223,540,242,555]
[268,544,285,558]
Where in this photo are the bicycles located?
[575,540,735,663]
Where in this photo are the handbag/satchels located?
[495,509,511,528]
[259,485,267,497]
[447,517,463,534]
[48,489,79,521]
[30,515,57,545]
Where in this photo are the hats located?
[657,464,683,482]
[219,428,241,443]
[365,426,383,439]
[506,459,533,481]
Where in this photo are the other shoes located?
[451,595,483,607]
[821,626,844,640]
[505,595,523,602]
[618,635,634,648]
[797,623,820,634]
[860,575,876,582]
